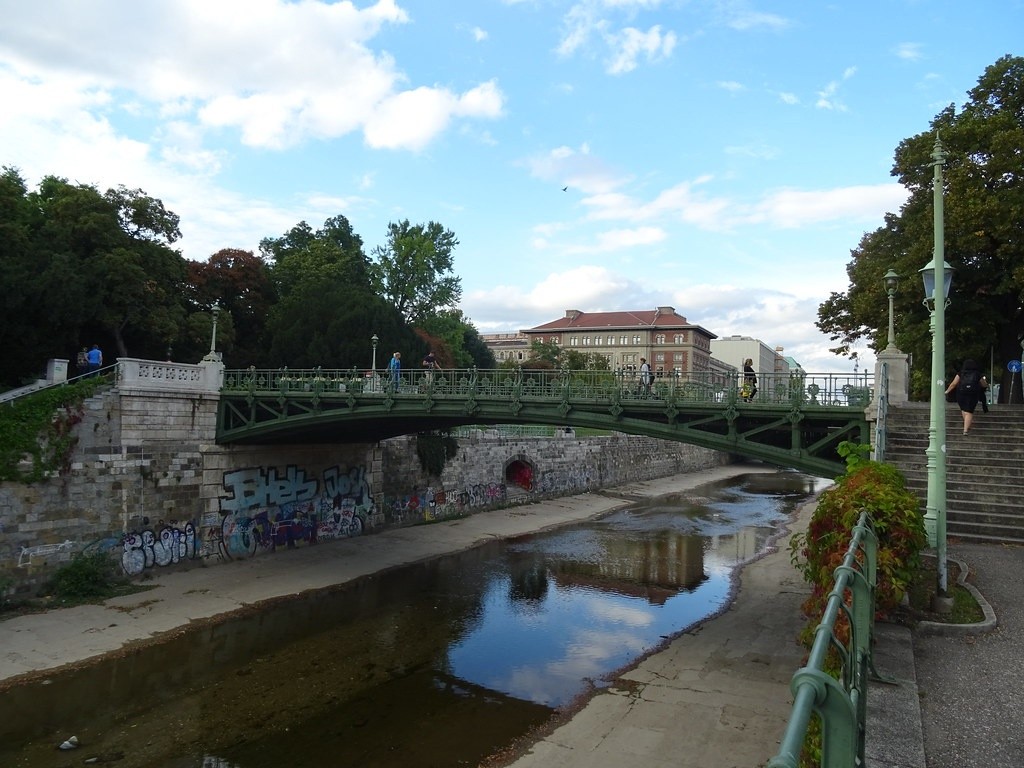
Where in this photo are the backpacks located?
[386,357,395,373]
[959,372,980,392]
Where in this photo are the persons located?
[384,352,401,394]
[423,351,442,390]
[744,359,757,403]
[945,359,989,435]
[76,347,88,379]
[634,358,659,400]
[85,345,102,378]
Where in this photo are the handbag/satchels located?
[742,379,753,397]
